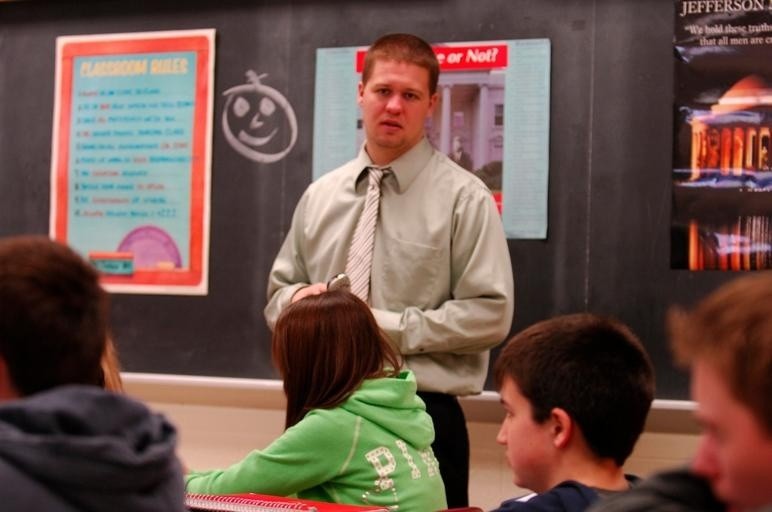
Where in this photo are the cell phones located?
[326,273,351,294]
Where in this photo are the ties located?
[344,166,392,304]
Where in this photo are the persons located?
[586,267,771,512]
[489,311,652,511]
[264,33,516,512]
[2,234,193,510]
[184,292,448,510]
